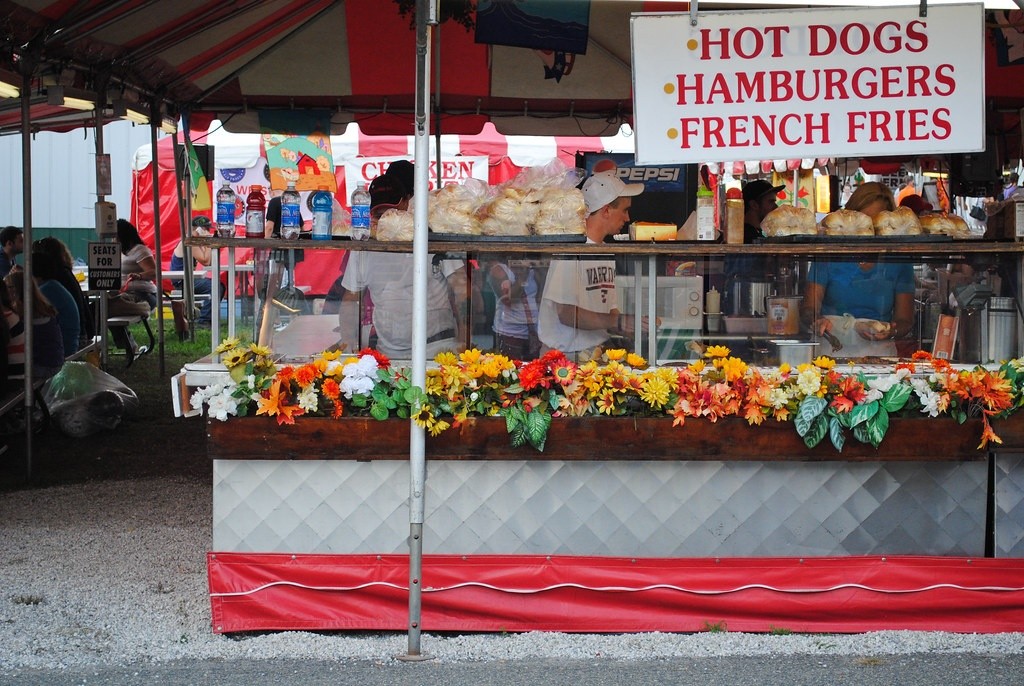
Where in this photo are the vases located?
[205,406,1024,461]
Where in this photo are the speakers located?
[180,143,215,181]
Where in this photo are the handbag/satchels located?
[529,331,542,357]
[108,277,152,318]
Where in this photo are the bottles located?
[311,185,333,240]
[216,180,237,238]
[696,190,716,242]
[705,285,720,313]
[350,181,371,242]
[724,188,744,246]
[280,182,300,240]
[246,185,266,238]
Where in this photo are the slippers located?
[125,345,148,368]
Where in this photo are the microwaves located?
[615,276,704,335]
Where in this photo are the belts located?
[427,329,455,344]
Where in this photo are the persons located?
[108,219,156,366]
[0,225,86,455]
[265,185,306,271]
[170,216,225,329]
[341,160,1024,365]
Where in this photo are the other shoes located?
[198,322,211,330]
[0,440,8,454]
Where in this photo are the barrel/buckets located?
[963,292,1015,363]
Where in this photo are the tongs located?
[823,330,843,350]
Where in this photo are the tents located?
[129,126,635,299]
[0,1,1024,661]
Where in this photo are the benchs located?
[162,294,211,301]
[162,299,205,307]
[107,316,143,326]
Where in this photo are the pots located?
[724,277,777,316]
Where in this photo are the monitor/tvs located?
[582,151,698,235]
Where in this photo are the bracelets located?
[891,328,898,339]
[139,273,142,280]
[618,314,621,330]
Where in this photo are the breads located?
[332,182,587,243]
[762,206,969,235]
[854,320,891,332]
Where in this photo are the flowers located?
[190,335,1024,455]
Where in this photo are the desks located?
[203,263,253,326]
[162,270,207,342]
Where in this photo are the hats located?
[899,194,933,215]
[193,216,216,228]
[742,180,786,203]
[369,175,406,213]
[582,172,644,213]
